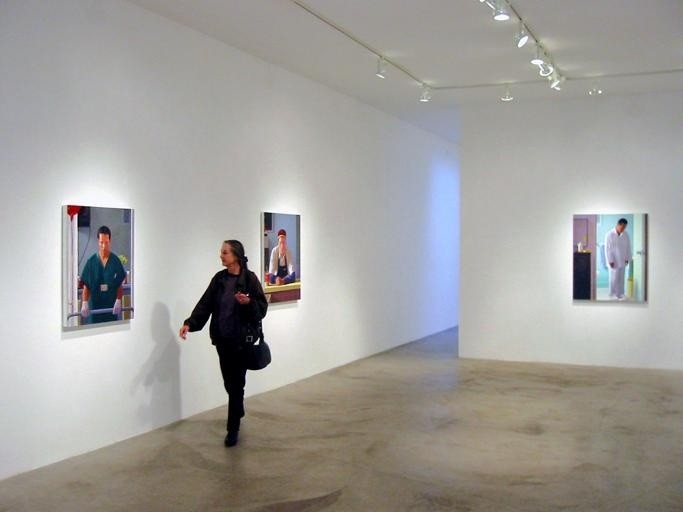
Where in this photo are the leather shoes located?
[222,430,240,448]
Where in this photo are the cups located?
[265,274,270,286]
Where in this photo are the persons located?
[268,229,296,286]
[179,240,268,447]
[80,226,127,324]
[604,218,632,299]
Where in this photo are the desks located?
[263,276,301,302]
[75,282,131,300]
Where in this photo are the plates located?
[583,249,591,253]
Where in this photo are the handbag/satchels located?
[245,337,274,372]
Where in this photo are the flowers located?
[117,253,128,265]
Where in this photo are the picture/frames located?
[573,217,589,248]
[119,209,130,226]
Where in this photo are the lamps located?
[374,54,431,105]
[498,73,604,104]
[473,0,567,92]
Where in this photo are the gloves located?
[81,300,91,318]
[112,298,122,315]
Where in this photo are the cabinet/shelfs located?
[573,250,592,299]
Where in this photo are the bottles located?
[577,242,583,252]
